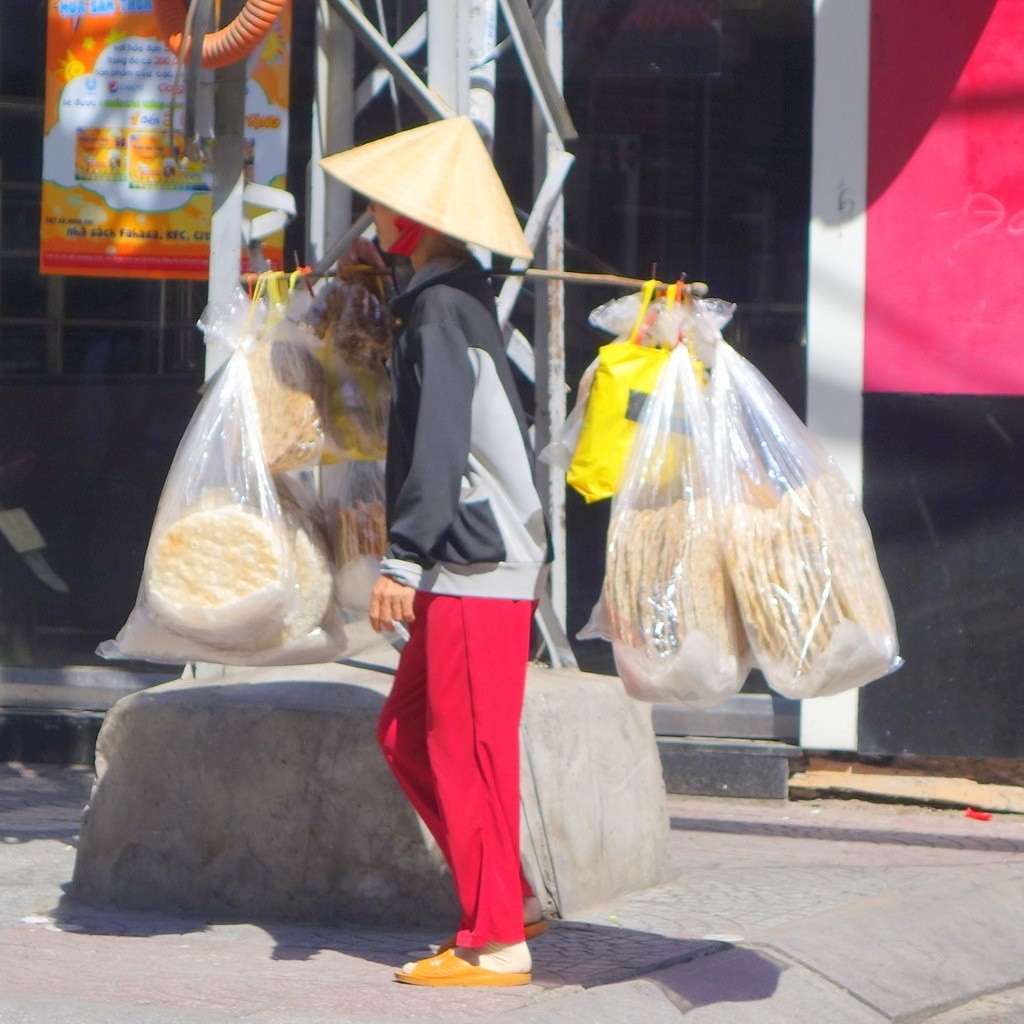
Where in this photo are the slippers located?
[429,903,549,958]
[394,946,531,988]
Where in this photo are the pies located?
[146,344,386,643]
[607,476,894,675]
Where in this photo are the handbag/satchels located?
[247,267,394,463]
[566,279,712,504]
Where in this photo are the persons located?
[316,113,548,986]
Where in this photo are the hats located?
[319,112,534,260]
